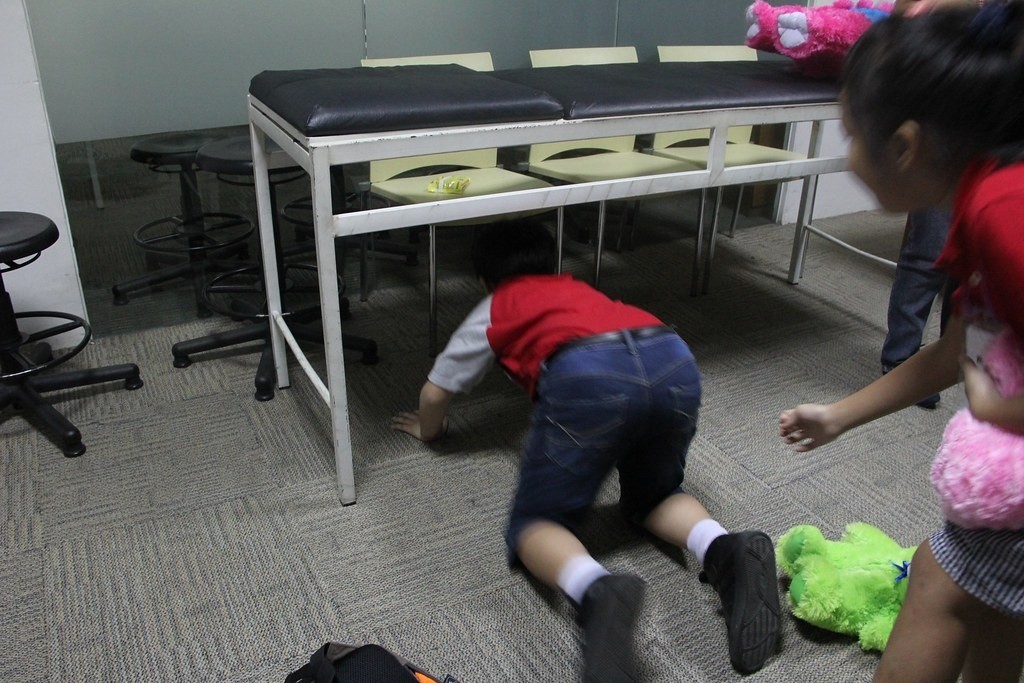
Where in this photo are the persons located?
[391,220,784,683]
[779,1,1024,683]
[881,199,962,407]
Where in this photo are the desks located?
[247,61,844,508]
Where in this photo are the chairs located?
[529,46,705,297]
[359,52,565,357]
[625,45,811,271]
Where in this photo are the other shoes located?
[882,371,940,407]
[575,574,647,683]
[698,531,782,676]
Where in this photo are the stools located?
[1,211,144,459]
[112,134,265,305]
[280,164,419,280]
[171,134,380,402]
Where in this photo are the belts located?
[545,326,675,365]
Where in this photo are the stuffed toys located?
[745,0,892,76]
[931,328,1024,530]
